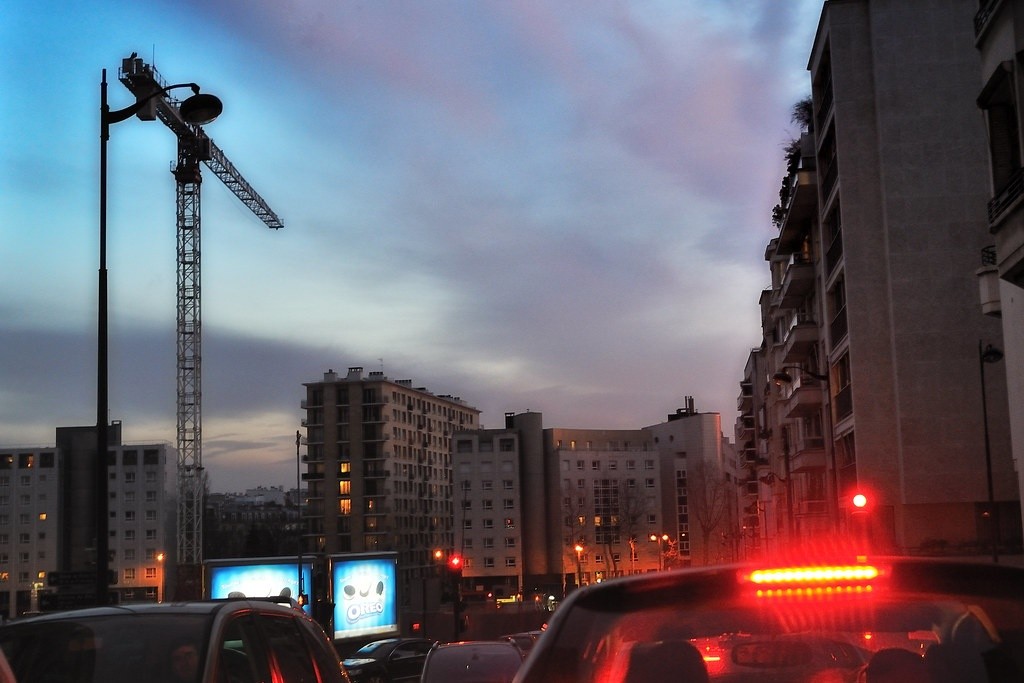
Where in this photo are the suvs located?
[509,555,1024,683]
[421,637,523,683]
[0,597,352,683]
[342,638,434,683]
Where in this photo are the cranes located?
[119,45,285,600]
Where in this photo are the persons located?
[167,636,203,683]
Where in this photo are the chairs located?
[220,648,253,683]
[866,648,930,683]
[623,640,710,683]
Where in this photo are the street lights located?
[760,471,794,553]
[97,69,223,604]
[774,357,841,549]
[651,533,668,571]
[575,543,583,589]
[748,502,768,558]
[978,338,1004,561]
